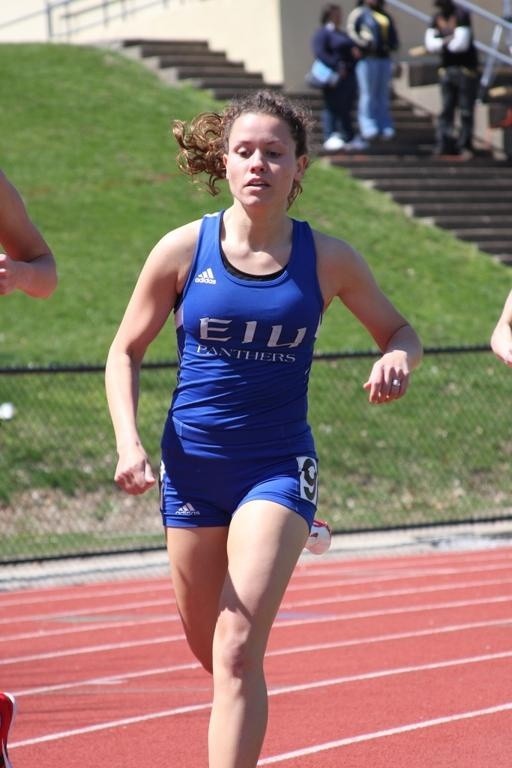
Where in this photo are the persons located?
[347,0,399,141]
[103,84,425,763]
[0,174,58,767]
[491,289,512,368]
[310,2,371,152]
[423,0,482,161]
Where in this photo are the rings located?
[392,379,401,386]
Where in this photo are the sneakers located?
[305,518,331,554]
[0,691,16,767]
[323,123,394,152]
[432,141,471,156]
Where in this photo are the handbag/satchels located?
[304,58,340,90]
